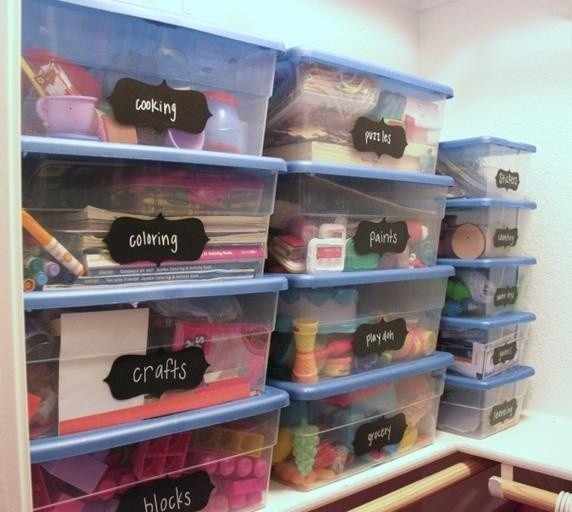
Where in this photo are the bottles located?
[202,90,247,153]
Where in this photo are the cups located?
[37,96,98,135]
[292,318,323,384]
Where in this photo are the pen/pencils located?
[22,208,85,292]
